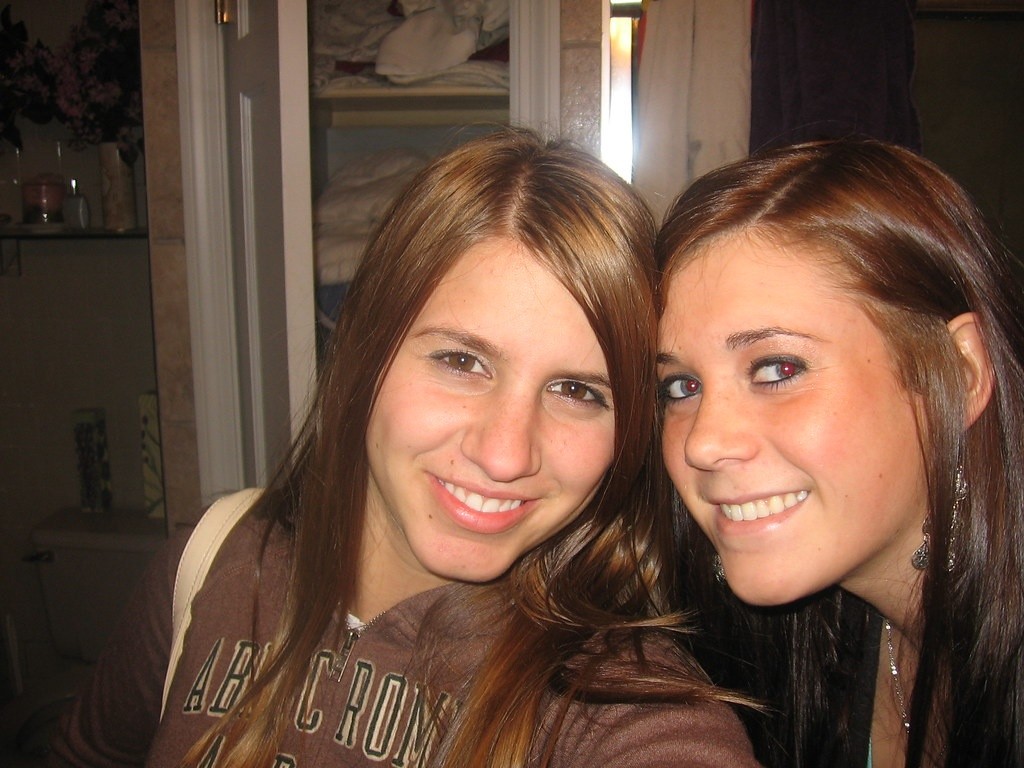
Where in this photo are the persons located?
[655,124,1024,768]
[42,122,764,768]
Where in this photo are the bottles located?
[66,180,88,232]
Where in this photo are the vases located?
[99,142,137,230]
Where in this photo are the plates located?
[17,222,66,233]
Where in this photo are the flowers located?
[1,0,144,168]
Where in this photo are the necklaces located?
[887,620,948,743]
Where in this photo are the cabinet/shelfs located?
[175,0,561,507]
